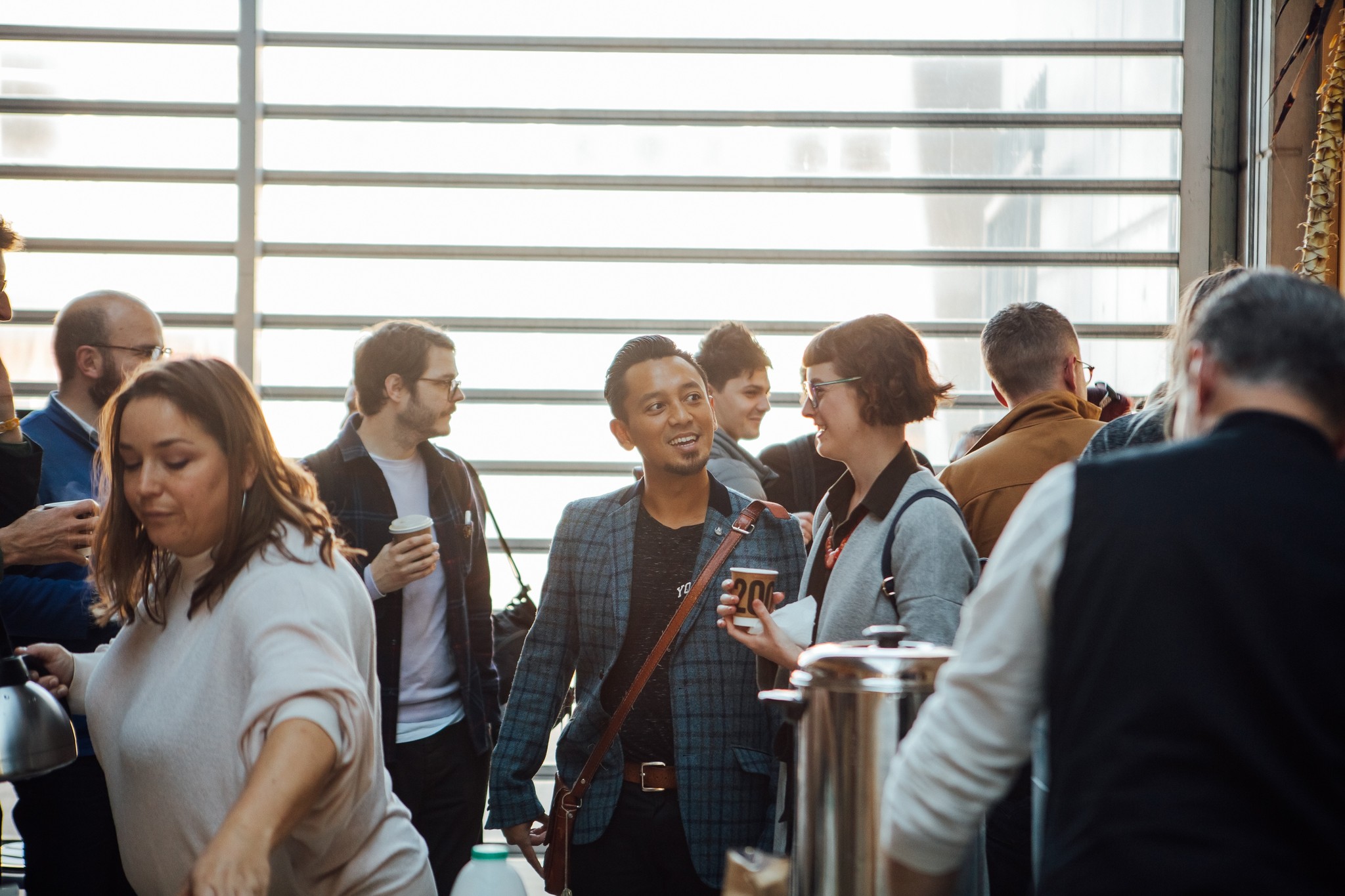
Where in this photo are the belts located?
[624,761,677,793]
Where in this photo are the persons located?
[714,312,984,896]
[13,353,441,896]
[479,333,807,896]
[695,317,782,501]
[294,317,502,896]
[936,299,1113,568]
[0,210,1345,896]
[876,268,1344,895]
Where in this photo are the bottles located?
[449,843,527,896]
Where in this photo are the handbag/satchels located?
[543,770,582,896]
[492,584,575,731]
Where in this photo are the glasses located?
[414,376,462,402]
[92,344,173,364]
[1064,358,1095,386]
[1095,382,1121,412]
[803,375,863,409]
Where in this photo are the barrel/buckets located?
[758,622,992,896]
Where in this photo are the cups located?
[730,566,779,629]
[389,514,434,544]
[44,500,93,558]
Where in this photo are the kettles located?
[0,653,79,781]
